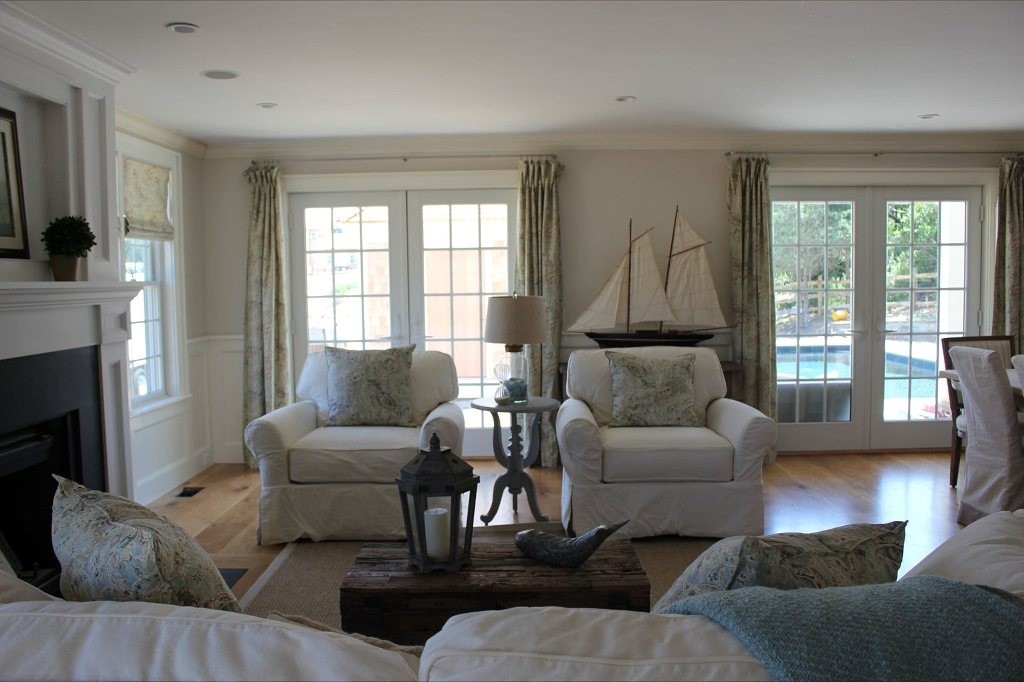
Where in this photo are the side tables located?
[470,393,562,529]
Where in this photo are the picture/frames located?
[0,106,33,260]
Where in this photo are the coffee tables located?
[336,540,652,643]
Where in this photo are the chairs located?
[941,335,1021,489]
[944,346,1023,526]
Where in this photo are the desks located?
[939,367,1023,403]
[559,358,744,406]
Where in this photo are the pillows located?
[653,518,909,616]
[602,349,702,428]
[49,471,245,616]
[324,344,422,430]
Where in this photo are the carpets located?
[231,521,724,640]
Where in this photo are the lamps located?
[483,290,551,406]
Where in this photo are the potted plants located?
[39,214,99,282]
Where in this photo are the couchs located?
[0,504,1024,682]
[241,347,466,549]
[555,345,778,542]
